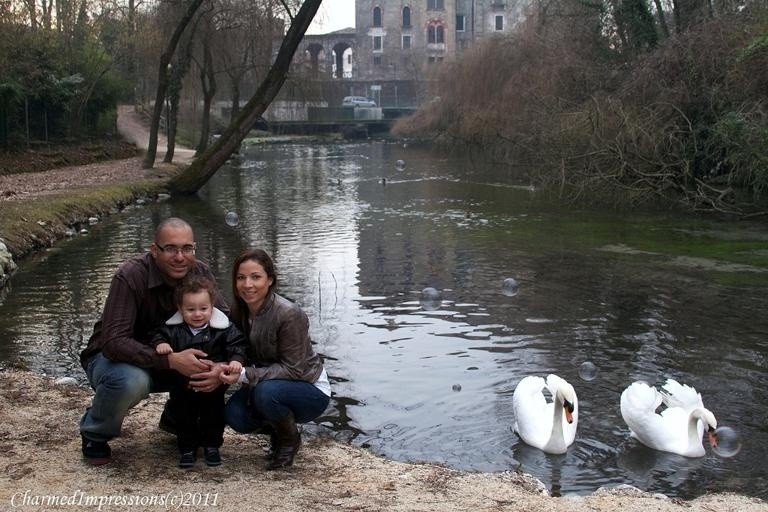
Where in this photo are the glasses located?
[155,242,195,252]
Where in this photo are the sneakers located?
[82,435,111,465]
[179,448,221,467]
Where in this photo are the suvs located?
[342,96,377,109]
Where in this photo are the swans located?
[620,377,718,459]
[512,373,579,455]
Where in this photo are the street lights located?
[164,62,174,159]
[386,59,399,108]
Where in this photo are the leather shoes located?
[268,440,300,468]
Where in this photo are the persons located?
[216,247,332,470]
[147,273,250,468]
[77,216,233,466]
